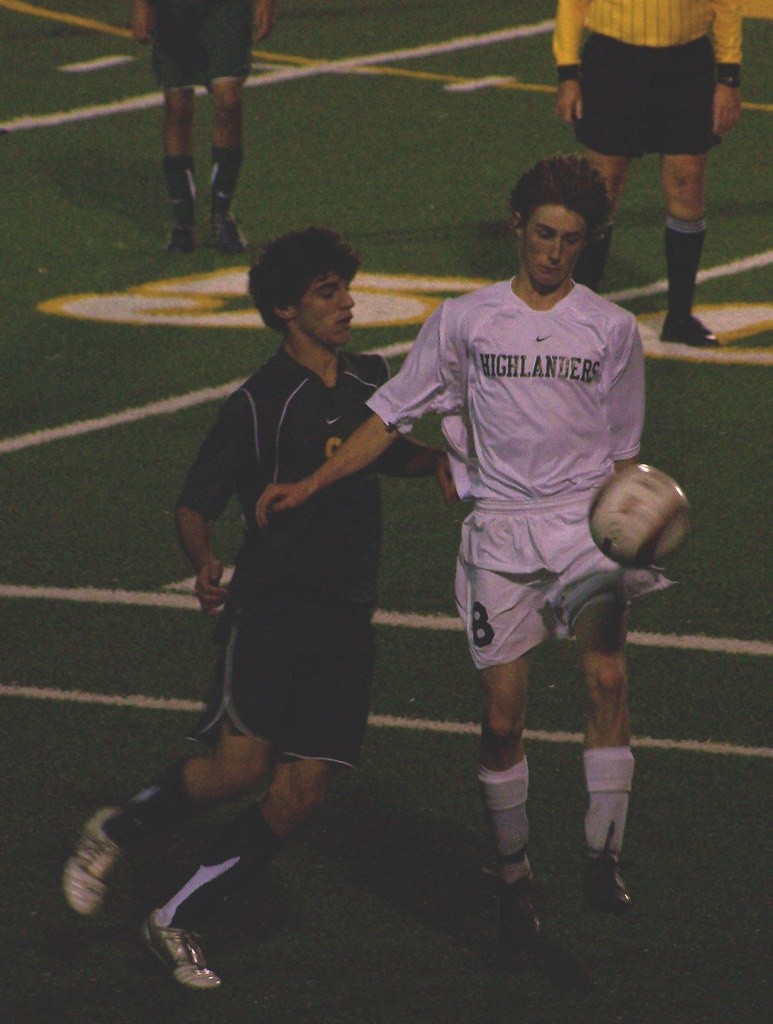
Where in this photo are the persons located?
[63,231,456,989]
[133,0,273,255]
[552,0,743,348]
[256,157,645,934]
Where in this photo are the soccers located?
[586,461,691,566]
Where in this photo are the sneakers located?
[491,869,542,943]
[578,820,632,912]
[62,804,124,917]
[140,907,222,991]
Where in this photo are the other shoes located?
[166,223,198,257]
[209,209,247,253]
[661,314,720,347]
